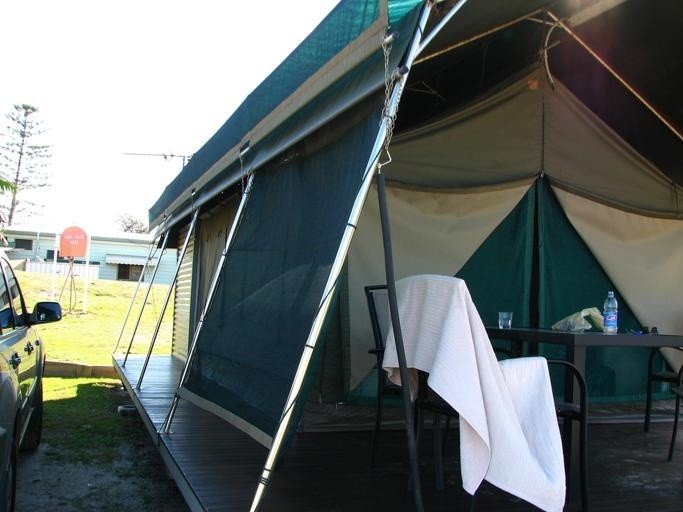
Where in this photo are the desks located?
[483,322,682,512]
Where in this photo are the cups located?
[499,312,512,329]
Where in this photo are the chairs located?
[643,346,683,463]
[402,275,592,512]
[365,283,409,458]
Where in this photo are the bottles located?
[603,291,617,335]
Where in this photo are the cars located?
[0,249,62,512]
